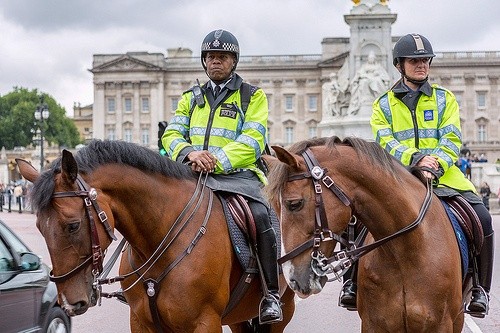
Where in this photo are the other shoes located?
[468,292,489,318]
[260,293,283,324]
[341,281,358,311]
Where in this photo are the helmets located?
[201,29,240,61]
[392,33,436,67]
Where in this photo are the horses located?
[14,139,296,333]
[260,136,464,333]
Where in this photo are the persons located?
[0,180,35,209]
[340,32,489,318]
[480,180,492,211]
[326,72,340,98]
[328,94,340,117]
[161,29,284,323]
[353,50,390,99]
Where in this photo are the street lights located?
[34,103,50,172]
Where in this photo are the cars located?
[0,217,73,333]
